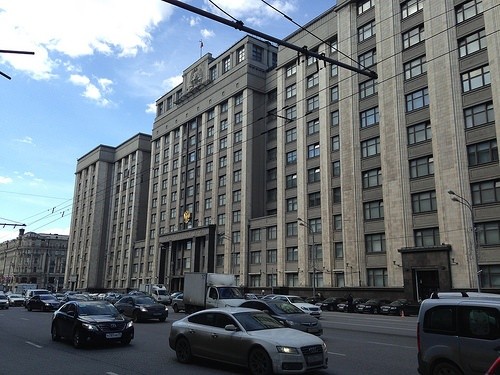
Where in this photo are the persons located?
[347,293,353,313]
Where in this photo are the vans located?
[25,288,51,301]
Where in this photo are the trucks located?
[139,283,171,304]
[11,282,38,295]
[183,272,248,314]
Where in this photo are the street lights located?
[447,189,481,294]
[218,232,236,274]
[296,217,316,297]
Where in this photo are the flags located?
[201,41,203,47]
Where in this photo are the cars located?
[0,291,26,309]
[168,307,329,375]
[50,290,169,349]
[237,299,324,336]
[245,293,421,320]
[169,292,186,313]
[26,294,61,313]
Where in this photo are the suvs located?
[417,292,500,375]
